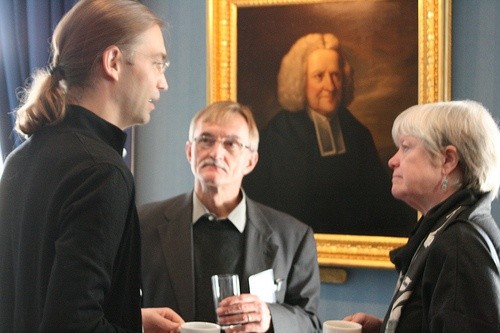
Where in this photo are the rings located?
[247,313,251,323]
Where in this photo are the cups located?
[323,320,362,333]
[211,274,242,329]
[176,321,221,333]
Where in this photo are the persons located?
[135,101,323,333]
[0,0,185,333]
[342,99,500,333]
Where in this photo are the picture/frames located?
[206,0,452,268]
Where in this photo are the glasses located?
[191,135,255,154]
[120,47,169,72]
